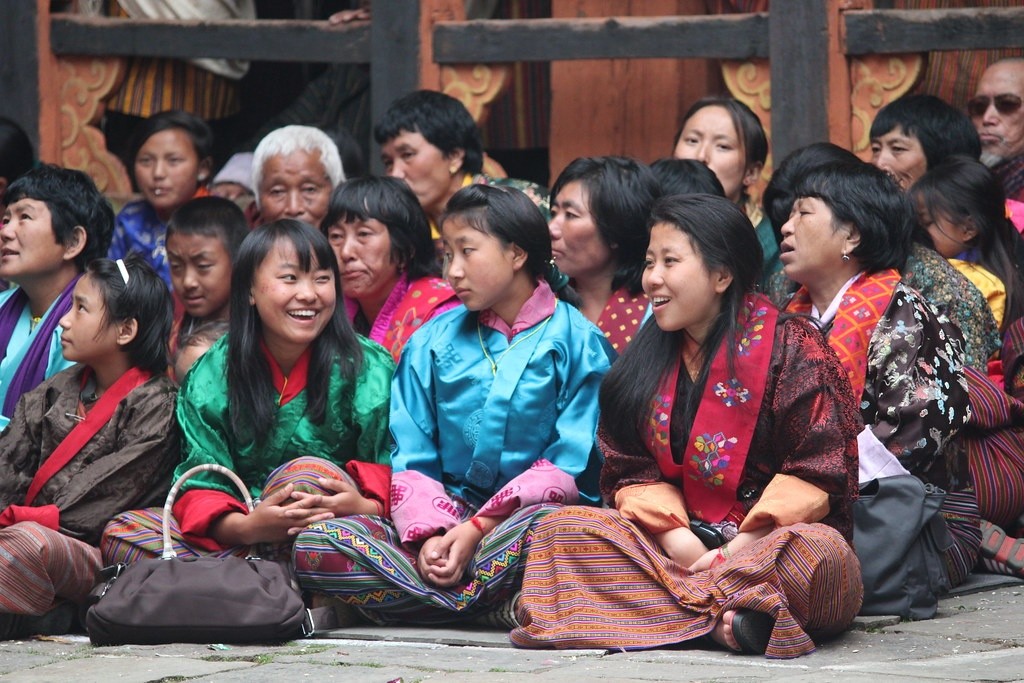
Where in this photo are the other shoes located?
[974,519,1024,578]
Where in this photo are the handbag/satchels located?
[849,475,956,622]
[0,505,61,531]
[87,461,306,645]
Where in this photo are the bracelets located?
[469,516,486,536]
[367,498,381,516]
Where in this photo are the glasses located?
[968,93,1022,117]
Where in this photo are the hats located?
[208,151,260,195]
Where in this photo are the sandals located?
[730,609,775,655]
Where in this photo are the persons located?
[0,162,118,429]
[106,110,219,293]
[509,196,863,660]
[244,0,374,184]
[758,57,1024,622]
[666,94,781,286]
[0,251,179,642]
[249,124,348,233]
[543,145,657,357]
[162,195,251,385]
[374,88,575,288]
[1,116,38,197]
[97,217,399,629]
[293,182,620,630]
[644,157,727,218]
[318,173,467,366]
[101,0,376,176]
